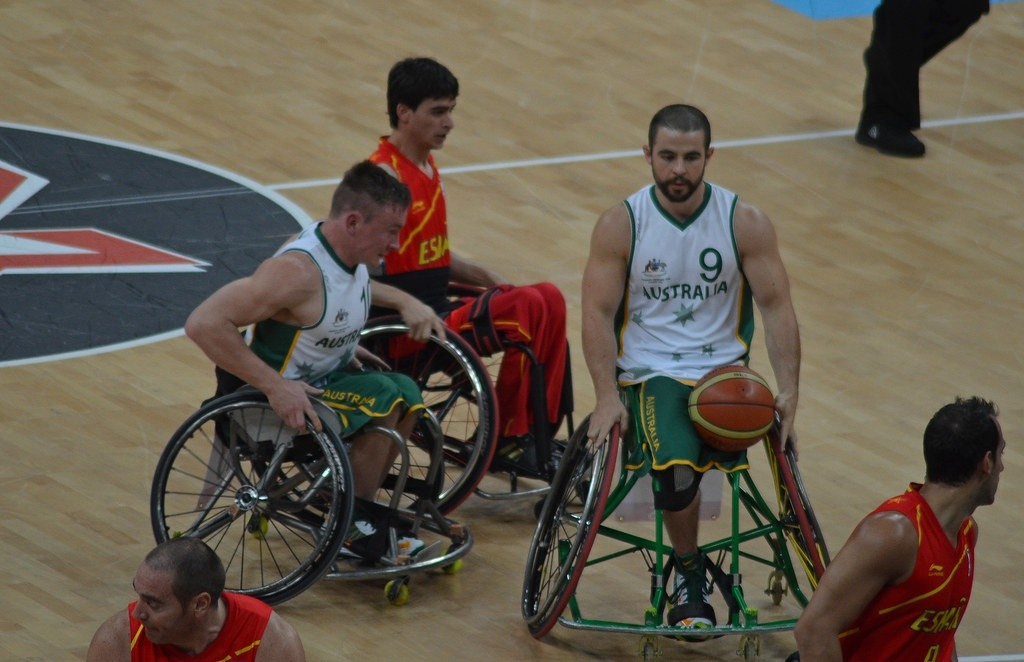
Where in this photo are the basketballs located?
[688,365,775,450]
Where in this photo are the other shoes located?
[855,120,925,158]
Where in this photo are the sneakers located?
[672,546,712,642]
[472,426,539,474]
[325,521,424,562]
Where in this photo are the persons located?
[854,0,991,160]
[786,396,1006,662]
[355,58,572,480]
[184,159,426,566]
[87,537,306,662]
[581,104,802,644]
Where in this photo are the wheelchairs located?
[359,256,599,520]
[521,410,831,662]
[149,329,474,607]
